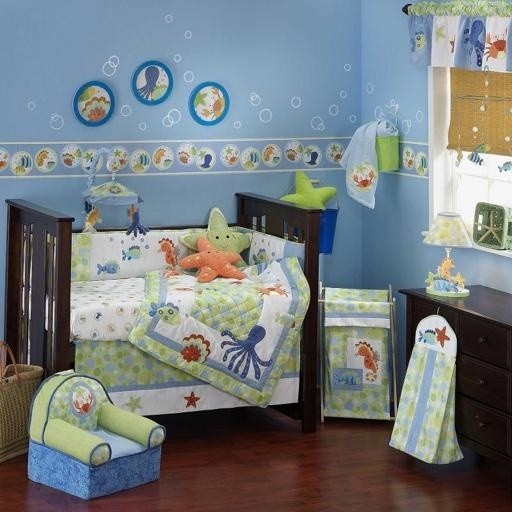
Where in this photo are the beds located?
[6,192,321,434]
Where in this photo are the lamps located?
[422,211,472,297]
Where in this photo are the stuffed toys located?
[125,211,149,238]
[84,207,102,235]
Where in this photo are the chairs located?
[27,369,166,500]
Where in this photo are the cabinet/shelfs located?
[398,284,512,466]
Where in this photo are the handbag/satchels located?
[0,341,45,462]
